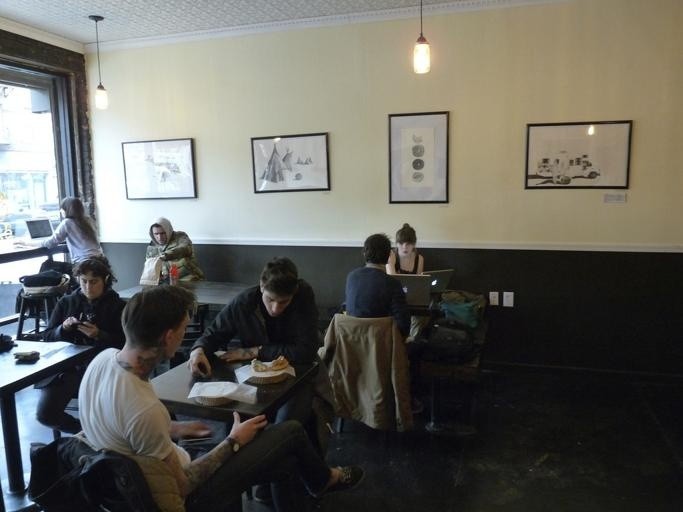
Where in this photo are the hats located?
[396,223,416,243]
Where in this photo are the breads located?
[272,355,289,371]
[252,358,267,371]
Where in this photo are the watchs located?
[226,435,240,453]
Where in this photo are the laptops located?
[388,274,431,307]
[421,269,455,292]
[24,218,54,240]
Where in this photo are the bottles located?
[170,263,179,287]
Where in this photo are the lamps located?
[86,13,113,109]
[412,1,430,75]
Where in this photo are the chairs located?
[29,434,261,512]
[2,241,499,444]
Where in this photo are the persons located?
[385,223,424,275]
[183,256,322,506]
[342,233,412,348]
[18,197,104,288]
[72,283,367,512]
[141,217,207,285]
[34,253,128,439]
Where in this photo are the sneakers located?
[411,397,425,415]
[319,465,366,497]
[253,483,272,503]
[425,419,478,437]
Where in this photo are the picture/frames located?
[250,131,332,192]
[523,120,633,188]
[120,135,197,201]
[387,110,450,204]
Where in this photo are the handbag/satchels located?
[58,312,106,375]
[19,269,63,287]
[429,324,475,365]
[403,289,489,351]
